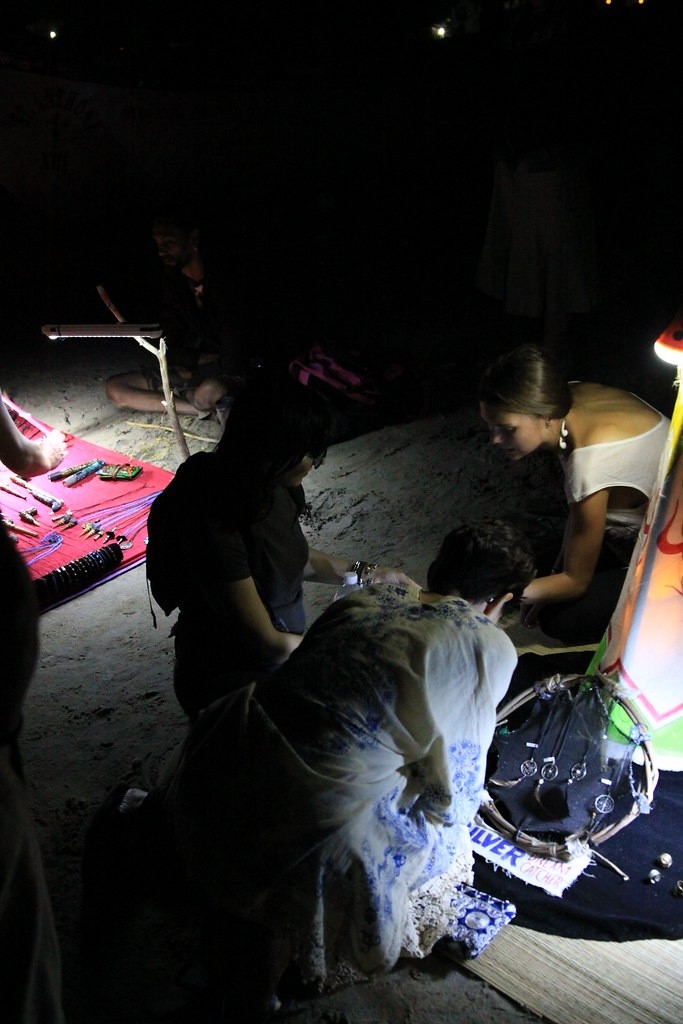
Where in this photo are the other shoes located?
[436,935,472,963]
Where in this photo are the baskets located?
[481,673,660,860]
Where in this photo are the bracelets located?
[353,561,377,588]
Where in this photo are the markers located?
[47,458,99,482]
[63,461,105,488]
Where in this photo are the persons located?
[476,341,671,645]
[103,207,270,422]
[242,515,535,994]
[0,392,81,1024]
[173,386,405,723]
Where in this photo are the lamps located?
[39,322,164,343]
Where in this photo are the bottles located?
[334,572,360,605]
